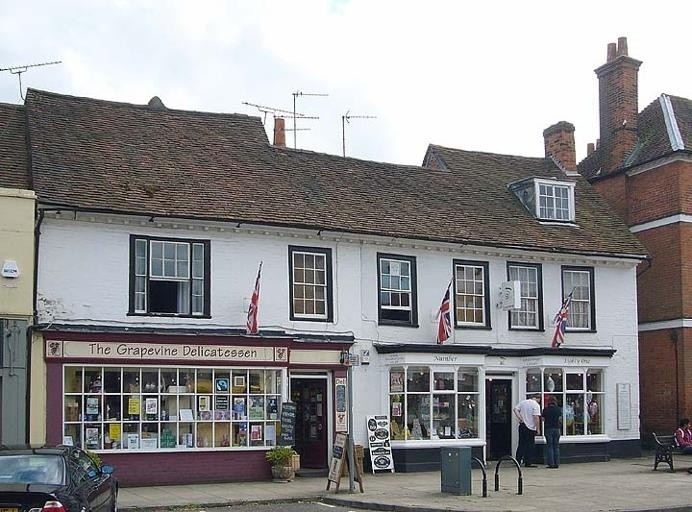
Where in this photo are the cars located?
[0,445,121,512]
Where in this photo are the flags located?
[248,267,262,336]
[436,284,451,344]
[552,292,573,348]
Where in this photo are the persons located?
[512,393,541,468]
[540,396,562,469]
[673,417,692,473]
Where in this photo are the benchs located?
[651,432,690,473]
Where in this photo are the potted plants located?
[265,447,296,483]
